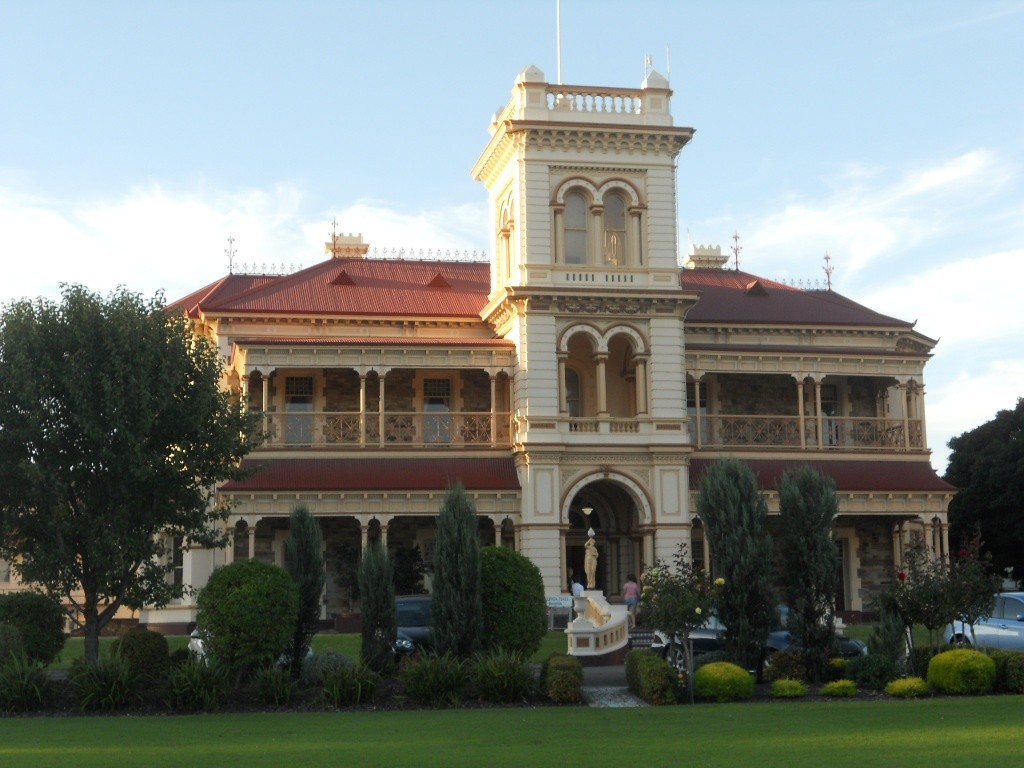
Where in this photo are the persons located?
[583,539,599,589]
[624,575,638,628]
[567,568,586,596]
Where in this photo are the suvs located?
[651,608,870,682]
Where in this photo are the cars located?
[942,592,1024,653]
[392,594,435,656]
